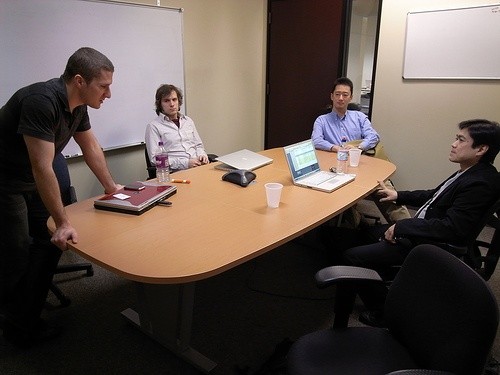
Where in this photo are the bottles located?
[336,139,350,175]
[155,141,170,183]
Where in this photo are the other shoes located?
[24,320,64,341]
[359,310,383,326]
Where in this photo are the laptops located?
[214,149,273,172]
[283,139,357,192]
[93,181,178,215]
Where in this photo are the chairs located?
[142,140,217,180]
[260,189,500,375]
[329,102,382,224]
[47,154,93,304]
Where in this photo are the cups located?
[349,149,362,167]
[264,183,283,208]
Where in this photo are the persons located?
[347,120,500,327]
[146,85,209,171]
[311,78,411,224]
[0,48,126,341]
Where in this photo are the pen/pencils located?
[171,178,190,183]
[158,200,172,205]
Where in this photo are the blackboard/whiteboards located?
[401,3,500,80]
[0,0,188,160]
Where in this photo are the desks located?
[48,145,398,374]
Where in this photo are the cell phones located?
[124,184,146,190]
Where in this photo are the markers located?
[66,153,78,157]
[141,142,145,143]
